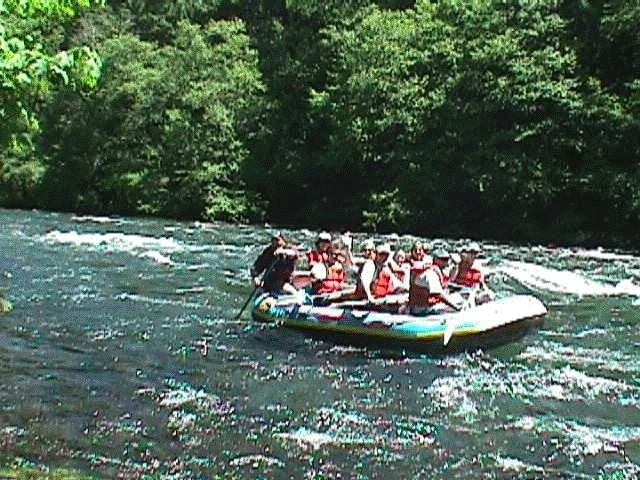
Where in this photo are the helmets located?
[432,248,450,259]
[319,232,331,242]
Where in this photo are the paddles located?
[443,275,483,345]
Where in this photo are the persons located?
[249,230,496,312]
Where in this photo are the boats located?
[251,287,546,355]
